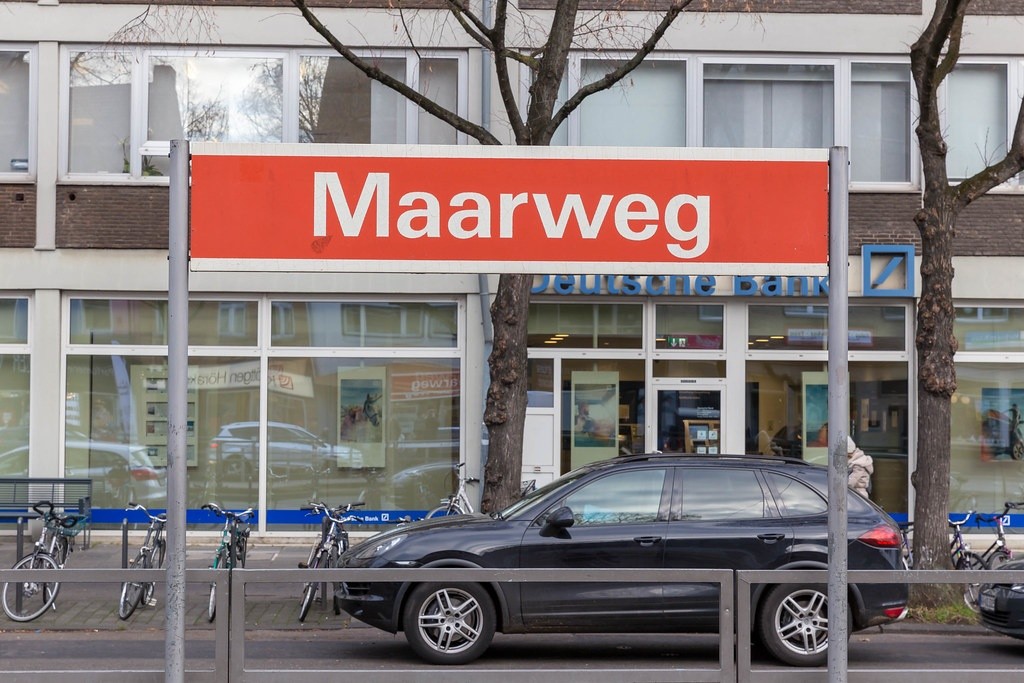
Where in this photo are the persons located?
[574,403,600,434]
[846,436,874,501]
[344,392,383,431]
[91,397,114,433]
[416,402,441,460]
[1004,402,1022,432]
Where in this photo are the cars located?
[980,410,1024,461]
[975,554,1024,641]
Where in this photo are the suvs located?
[208,421,362,482]
[359,459,458,515]
[332,453,912,668]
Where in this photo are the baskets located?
[44,511,88,537]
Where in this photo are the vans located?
[0,440,166,509]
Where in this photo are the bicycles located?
[298,459,329,510]
[900,511,991,615]
[298,502,366,622]
[201,502,254,623]
[107,460,136,509]
[254,468,288,513]
[950,501,1024,599]
[2,500,88,623]
[426,463,538,520]
[118,502,168,620]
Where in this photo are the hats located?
[847,435,856,453]
